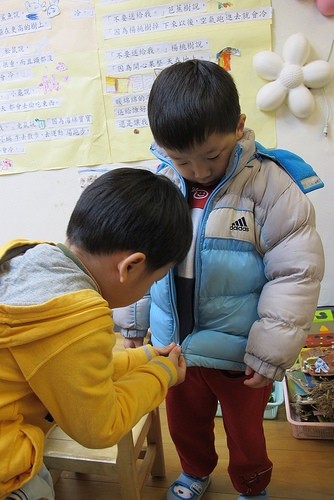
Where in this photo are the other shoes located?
[237,489,268,500]
[166,470,210,500]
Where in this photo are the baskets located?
[215,380,283,419]
[283,375,334,439]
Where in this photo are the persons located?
[0,167,193,500]
[108,59,324,500]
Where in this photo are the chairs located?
[43,407,166,500]
